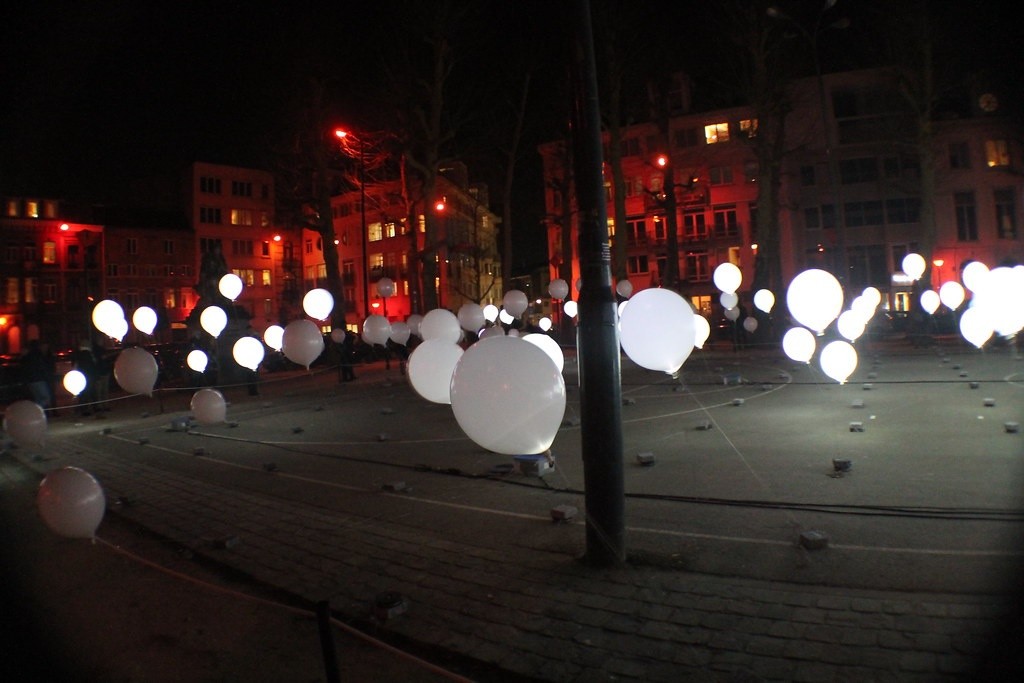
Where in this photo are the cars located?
[0,342,190,399]
[262,331,360,373]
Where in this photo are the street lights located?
[334,129,370,319]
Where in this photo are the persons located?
[29,341,62,418]
[339,319,358,381]
[134,394,149,417]
[78,334,112,416]
[248,372,260,396]
[204,243,228,274]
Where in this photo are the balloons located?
[616,279,710,379]
[712,263,882,385]
[362,277,581,406]
[92,274,345,397]
[901,253,925,281]
[920,261,1024,349]
[3,400,46,452]
[36,467,106,545]
[62,369,88,400]
[191,388,229,425]
[447,335,566,456]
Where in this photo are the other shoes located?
[83,412,91,416]
[346,376,355,382]
[106,408,112,412]
[53,412,60,416]
[96,408,103,413]
[248,392,262,398]
